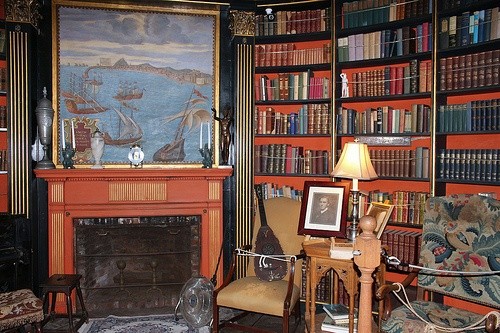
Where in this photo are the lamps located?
[331,142,380,250]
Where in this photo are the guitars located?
[254,184,288,281]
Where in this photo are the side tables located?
[304,238,386,333]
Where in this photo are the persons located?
[310,196,334,224]
[211,107,233,165]
[340,73,348,98]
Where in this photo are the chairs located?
[212,196,304,333]
[0,290,47,333]
[376,193,500,333]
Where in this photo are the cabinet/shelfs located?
[0,52,8,213]
[251,0,500,312]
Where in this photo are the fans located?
[178,274,214,333]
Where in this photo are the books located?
[330,247,354,260]
[0,67,7,91]
[347,190,429,226]
[336,147,431,179]
[338,0,433,97]
[320,304,357,333]
[0,149,7,171]
[335,104,432,133]
[254,8,333,37]
[438,98,500,132]
[252,103,331,136]
[254,144,332,175]
[437,149,500,185]
[253,183,303,203]
[300,263,359,308]
[381,230,422,273]
[255,42,332,67]
[438,50,500,90]
[438,5,500,49]
[0,106,7,129]
[255,72,332,100]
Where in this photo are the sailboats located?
[152,85,209,162]
[103,103,143,145]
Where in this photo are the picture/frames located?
[52,0,221,168]
[361,202,395,238]
[298,180,349,240]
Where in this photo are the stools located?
[38,274,89,333]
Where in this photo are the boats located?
[61,69,111,115]
[112,82,144,101]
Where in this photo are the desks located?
[33,167,234,312]
